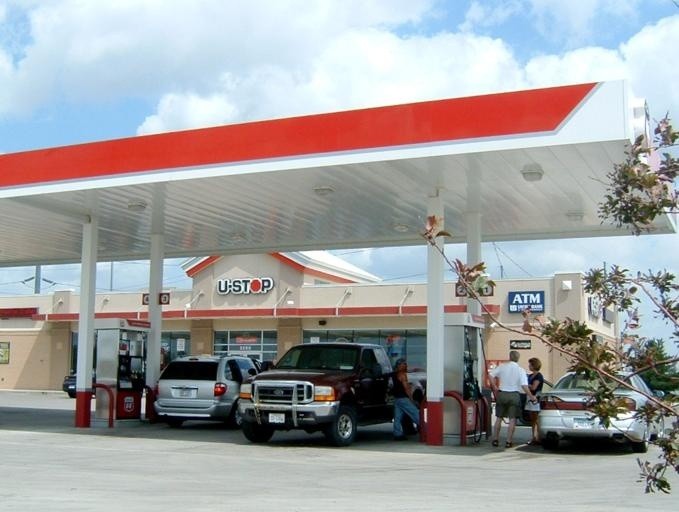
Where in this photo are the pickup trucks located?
[234,340,426,446]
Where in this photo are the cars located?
[63,365,97,399]
[512,369,665,452]
[153,354,264,429]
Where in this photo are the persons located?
[161,348,170,367]
[401,381,424,434]
[489,351,538,449]
[392,358,421,441]
[524,357,544,446]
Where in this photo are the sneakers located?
[528,440,541,446]
[392,435,408,440]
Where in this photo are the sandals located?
[505,442,512,448]
[492,440,498,447]
